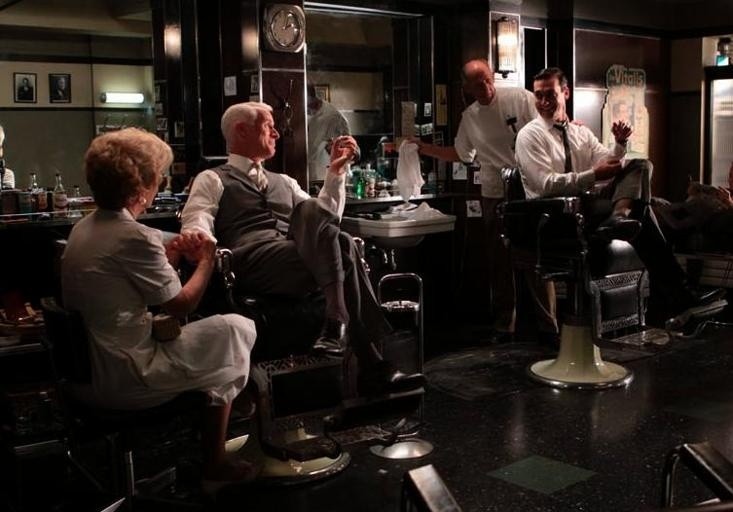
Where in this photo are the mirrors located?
[303,4,445,198]
[0,2,156,202]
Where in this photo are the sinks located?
[55,230,181,249]
[342,213,458,243]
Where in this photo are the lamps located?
[98,88,145,105]
[490,19,525,78]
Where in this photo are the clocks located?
[263,2,303,54]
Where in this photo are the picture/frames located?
[47,73,72,103]
[16,72,39,106]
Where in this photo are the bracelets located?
[615,139,627,146]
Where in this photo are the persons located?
[179,101,428,404]
[307,82,352,187]
[401,58,584,335]
[50,76,67,100]
[59,126,268,488]
[514,66,727,315]
[650,164,733,254]
[16,78,33,100]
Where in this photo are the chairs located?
[169,207,378,487]
[35,294,207,512]
[494,167,645,393]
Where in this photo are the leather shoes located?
[355,367,424,397]
[692,287,726,306]
[594,218,642,244]
[312,318,351,361]
[200,471,259,496]
[224,433,249,454]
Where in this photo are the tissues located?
[0,168,22,214]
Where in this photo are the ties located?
[253,160,268,190]
[552,120,571,174]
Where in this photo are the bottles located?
[361,164,375,198]
[27,172,37,191]
[72,184,81,197]
[355,180,365,200]
[51,173,67,218]
